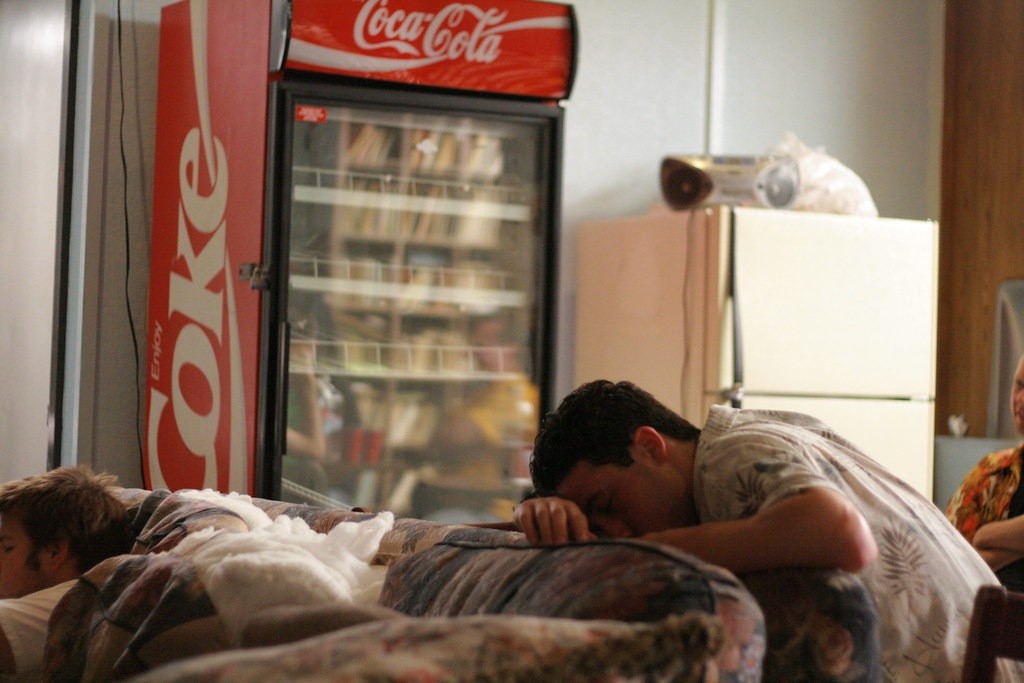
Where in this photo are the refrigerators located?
[573,208,939,504]
[143,0,581,533]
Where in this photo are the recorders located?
[659,153,800,211]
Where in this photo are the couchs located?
[39,479,767,683]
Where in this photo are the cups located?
[361,428,385,465]
[338,427,364,463]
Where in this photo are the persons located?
[946,357,1024,595]
[287,340,324,463]
[0,467,128,683]
[516,380,1024,683]
